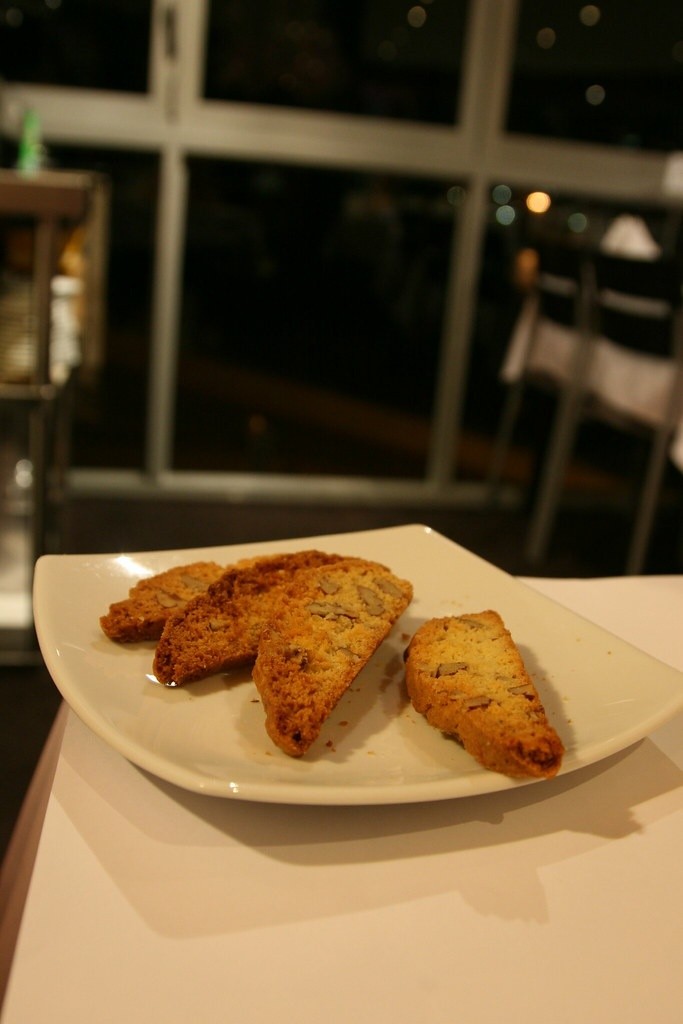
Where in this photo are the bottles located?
[15,110,50,173]
[47,275,88,385]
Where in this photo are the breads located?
[99,551,563,782]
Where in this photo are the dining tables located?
[1,576,683,1024]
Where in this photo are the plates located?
[30,522,683,807]
